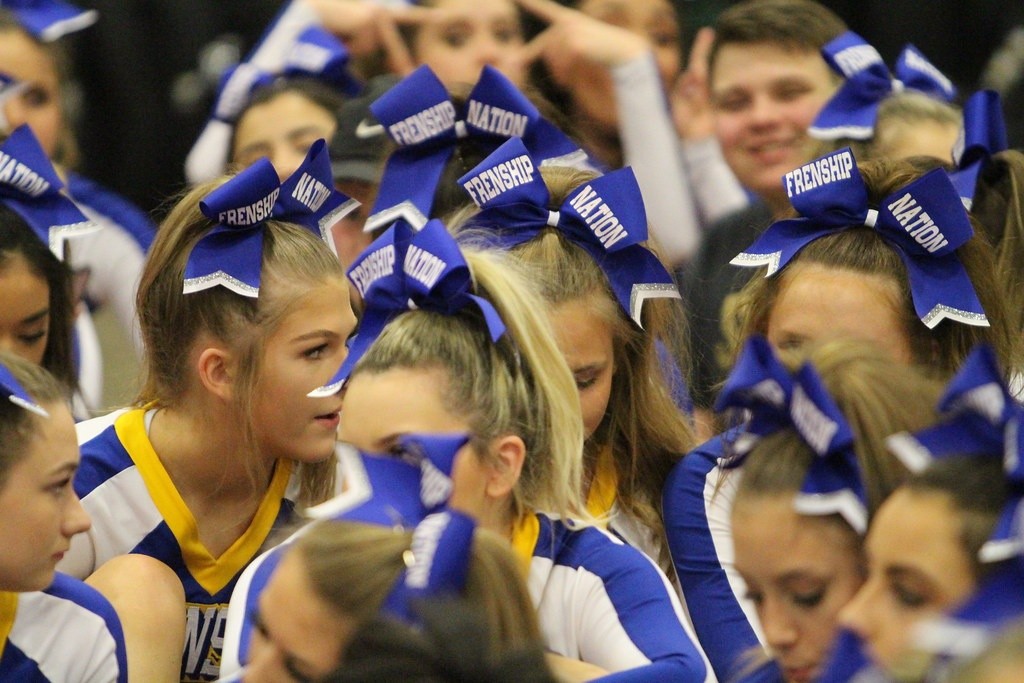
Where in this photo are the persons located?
[186,0,1024,437]
[662,148,1024,683]
[0,350,128,683]
[56,138,359,683]
[220,136,710,682]
[0,0,157,424]
